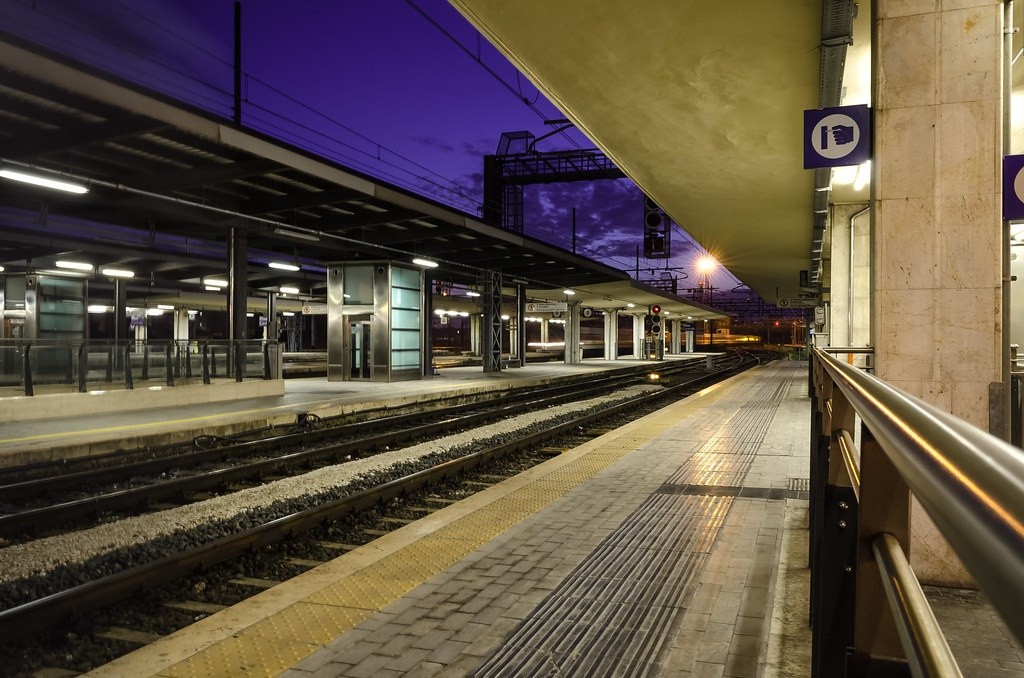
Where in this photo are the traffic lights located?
[643,193,665,229]
[650,302,662,334]
